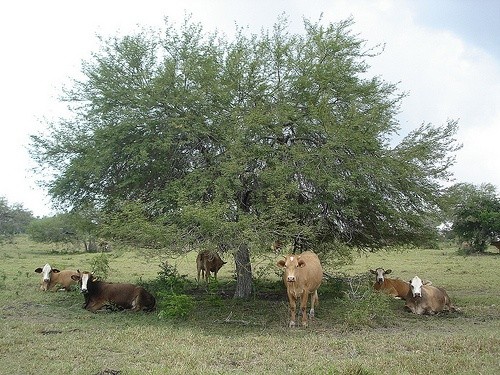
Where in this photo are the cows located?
[369,268,410,301]
[34,263,81,292]
[196,250,227,284]
[276,248,323,328]
[71,269,156,314]
[403,276,457,316]
